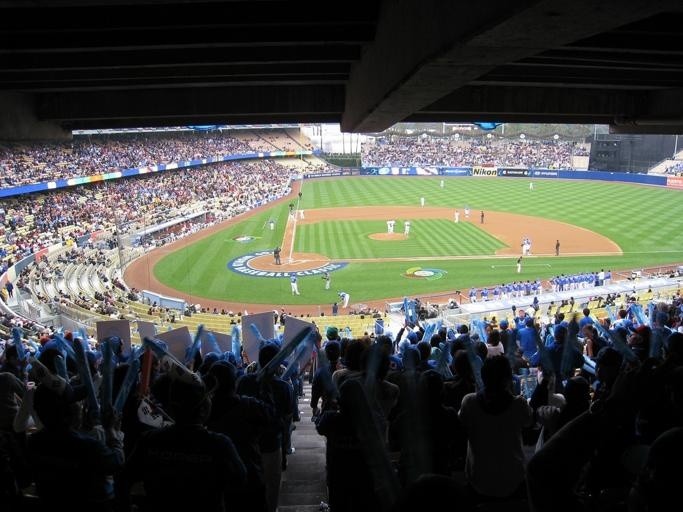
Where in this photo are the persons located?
[522,238,526,256]
[2,126,292,510]
[290,269,683,511]
[298,207,305,220]
[553,239,560,256]
[290,127,683,175]
[479,210,484,224]
[386,218,395,234]
[439,178,443,189]
[525,238,530,255]
[516,255,522,273]
[419,195,424,209]
[529,181,533,192]
[463,206,470,218]
[401,219,410,241]
[453,209,459,223]
[289,203,294,216]
[297,190,302,200]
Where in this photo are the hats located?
[591,346,623,369]
[629,324,652,337]
[327,327,338,335]
[33,375,92,418]
